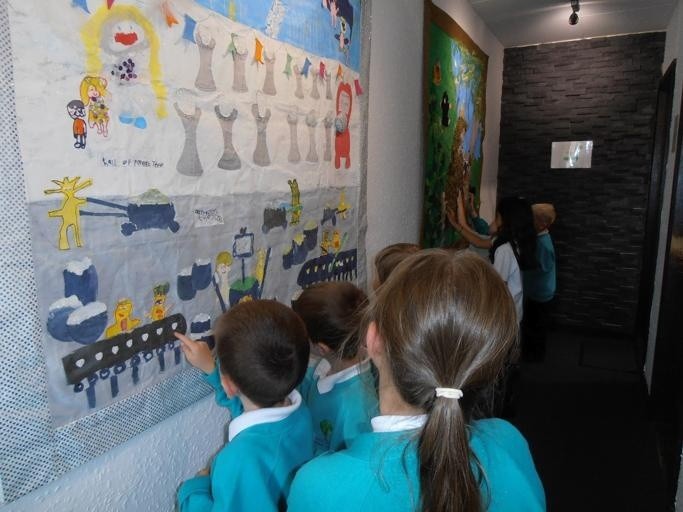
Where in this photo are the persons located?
[446,197,524,324]
[469,192,489,235]
[524,203,555,362]
[173,300,314,512]
[374,243,425,294]
[287,249,547,512]
[295,283,376,451]
[457,190,494,240]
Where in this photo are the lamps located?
[568,1,582,25]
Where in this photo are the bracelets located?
[457,225,463,234]
